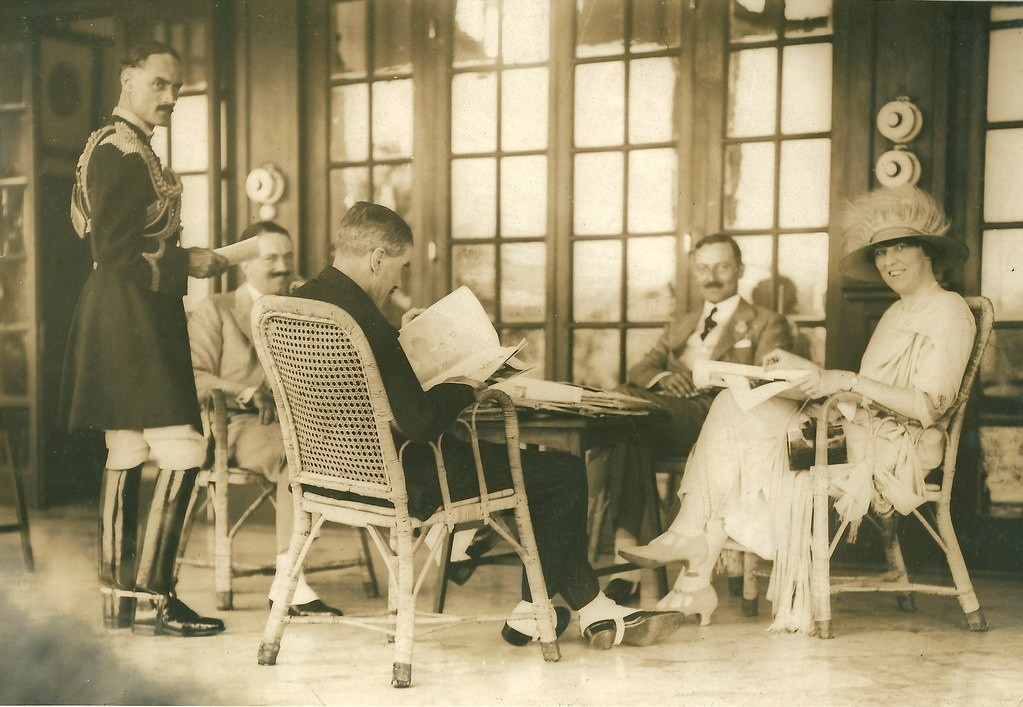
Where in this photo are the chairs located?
[742,295,995,640]
[589,315,799,597]
[250,294,560,685]
[170,389,380,609]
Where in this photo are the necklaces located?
[895,281,939,324]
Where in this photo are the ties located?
[699,305,718,341]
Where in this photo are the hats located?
[838,182,969,282]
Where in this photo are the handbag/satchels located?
[785,418,849,470]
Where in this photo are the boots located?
[97,461,149,629]
[130,466,226,638]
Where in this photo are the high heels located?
[618,528,709,576]
[652,584,719,626]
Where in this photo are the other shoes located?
[502,604,572,646]
[579,609,685,650]
[602,578,641,608]
[268,595,342,619]
[437,520,501,587]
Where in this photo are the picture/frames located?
[43,31,102,157]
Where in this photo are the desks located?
[431,403,668,623]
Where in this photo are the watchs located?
[849,370,859,392]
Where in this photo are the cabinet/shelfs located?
[0,0,47,510]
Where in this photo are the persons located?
[601,234,793,601]
[619,181,976,626]
[51,39,228,638]
[284,201,685,655]
[187,220,346,620]
